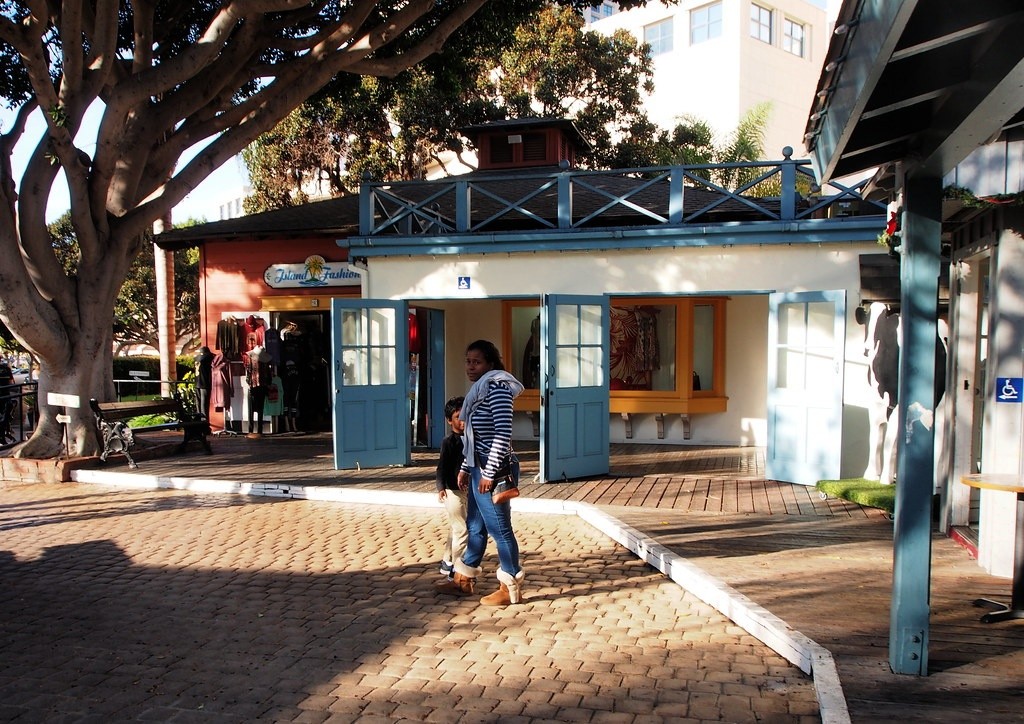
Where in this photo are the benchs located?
[89,393,214,469]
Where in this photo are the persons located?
[436,339,525,606]
[246,345,271,437]
[0,355,40,446]
[194,346,213,423]
[280,362,301,431]
[409,352,417,410]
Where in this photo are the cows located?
[855,303,950,483]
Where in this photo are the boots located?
[480,566,525,605]
[435,557,482,596]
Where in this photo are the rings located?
[485,488,490,492]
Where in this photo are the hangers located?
[284,320,301,336]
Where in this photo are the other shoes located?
[5,433,17,442]
[0,439,8,445]
[440,561,452,575]
[447,564,455,582]
[478,463,520,505]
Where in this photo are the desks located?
[960,473,1024,623]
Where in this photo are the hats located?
[245,345,270,362]
[196,347,211,362]
